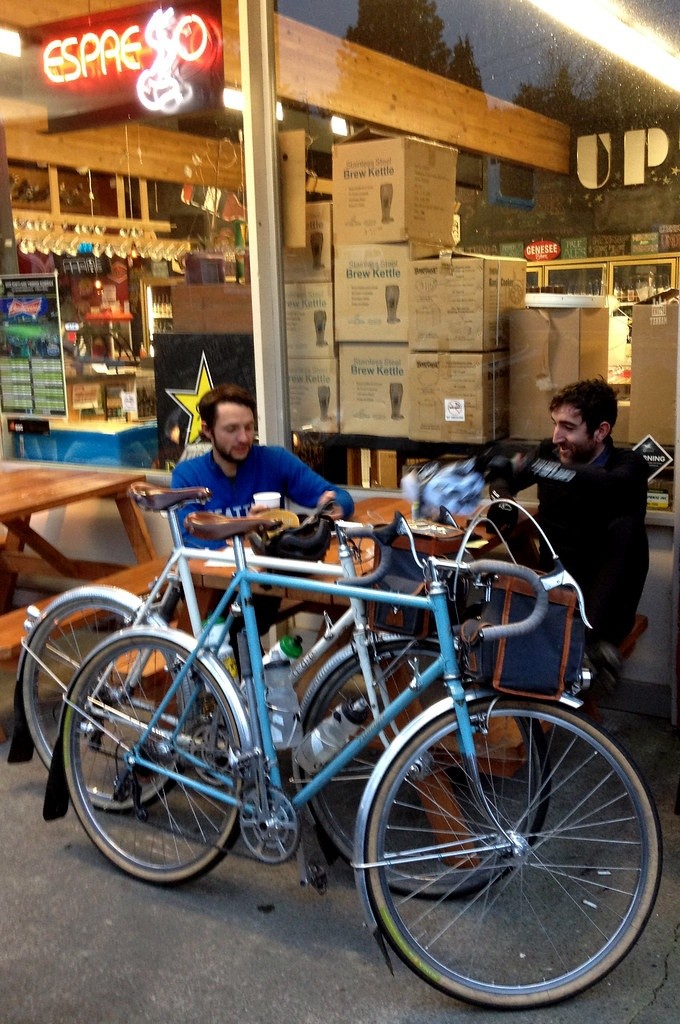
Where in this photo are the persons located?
[169,383,355,682]
[464,379,650,650]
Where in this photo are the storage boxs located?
[627,288,679,447]
[280,124,529,446]
[505,306,610,443]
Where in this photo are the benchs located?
[98,594,651,875]
[0,556,188,663]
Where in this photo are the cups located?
[379,183,394,224]
[102,285,116,307]
[313,310,326,346]
[317,385,330,420]
[390,383,404,419]
[253,492,281,510]
[384,285,400,324]
[310,231,324,270]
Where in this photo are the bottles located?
[297,698,368,776]
[263,650,302,750]
[154,319,168,334]
[613,280,670,302]
[203,613,239,689]
[238,633,304,703]
[179,183,245,222]
[139,344,146,360]
[153,294,172,314]
[566,279,600,296]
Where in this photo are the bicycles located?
[43,497,664,1012]
[7,481,549,897]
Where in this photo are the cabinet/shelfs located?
[526,252,680,306]
[140,275,187,363]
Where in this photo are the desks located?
[0,466,155,615]
[185,497,541,608]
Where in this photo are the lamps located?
[13,219,189,264]
